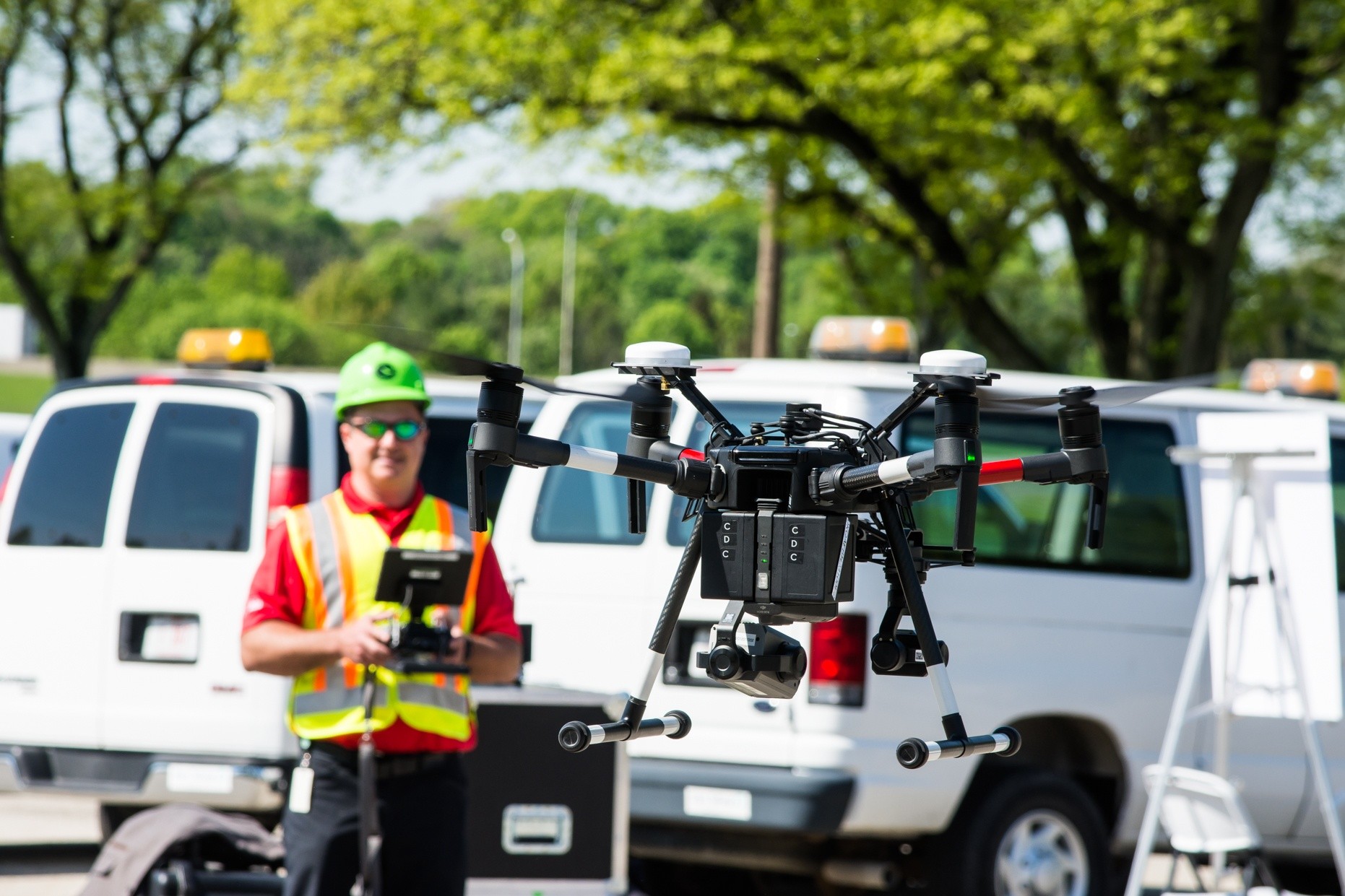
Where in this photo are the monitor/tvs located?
[375,547,475,606]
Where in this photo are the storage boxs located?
[461,685,630,895]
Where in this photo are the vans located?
[462,314,1345,896]
[0,324,570,896]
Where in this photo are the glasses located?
[345,419,429,441]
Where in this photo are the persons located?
[242,340,521,896]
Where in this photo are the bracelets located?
[463,638,473,660]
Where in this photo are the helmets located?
[332,341,431,422]
